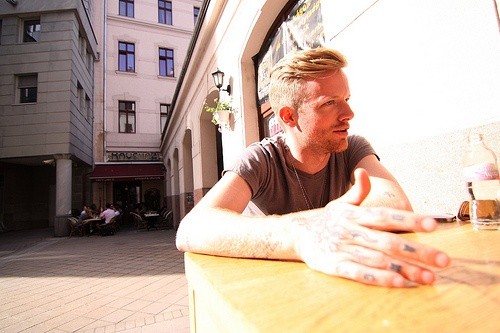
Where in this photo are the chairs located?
[130,206,173,232]
[67,211,124,239]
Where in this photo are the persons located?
[175,46,450,287]
[77,202,145,236]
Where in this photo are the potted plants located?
[204,97,236,134]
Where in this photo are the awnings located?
[89,161,164,179]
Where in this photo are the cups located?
[468,179,500,231]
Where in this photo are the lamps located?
[212,67,232,95]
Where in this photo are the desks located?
[184,220,500,333]
[145,214,159,231]
[88,219,101,236]
